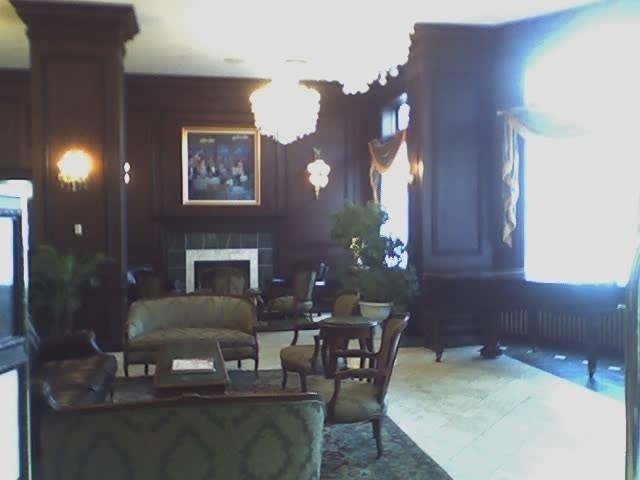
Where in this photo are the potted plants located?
[329,199,413,319]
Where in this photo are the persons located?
[188,143,252,200]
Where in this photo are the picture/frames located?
[181,125,262,207]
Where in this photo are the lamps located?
[305,146,332,201]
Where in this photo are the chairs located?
[258,268,315,326]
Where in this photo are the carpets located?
[257,311,319,332]
[104,369,453,480]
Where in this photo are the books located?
[171,356,215,374]
